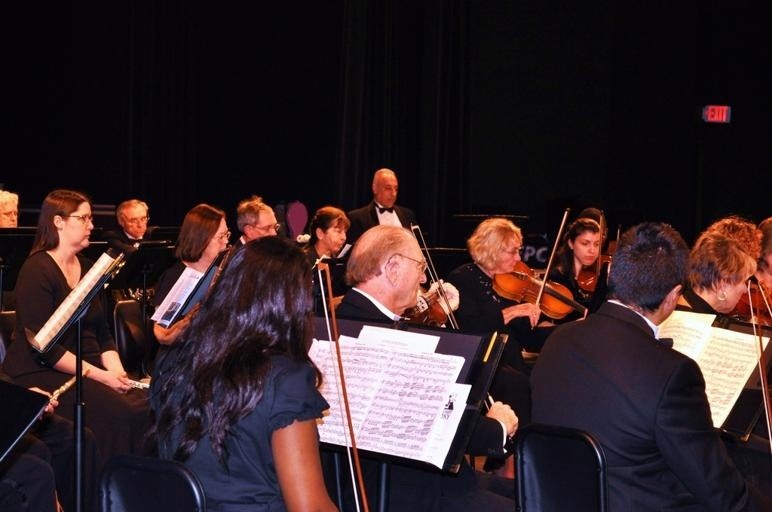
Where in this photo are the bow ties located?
[379,208,394,214]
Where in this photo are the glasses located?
[61,214,94,223]
[212,230,232,241]
[243,223,281,232]
[388,253,427,273]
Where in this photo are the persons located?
[1,168,771,512]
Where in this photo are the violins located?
[576,253,614,295]
[404,287,448,328]
[494,260,588,320]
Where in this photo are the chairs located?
[512,423,609,511]
[96,457,208,512]
[113,296,162,373]
